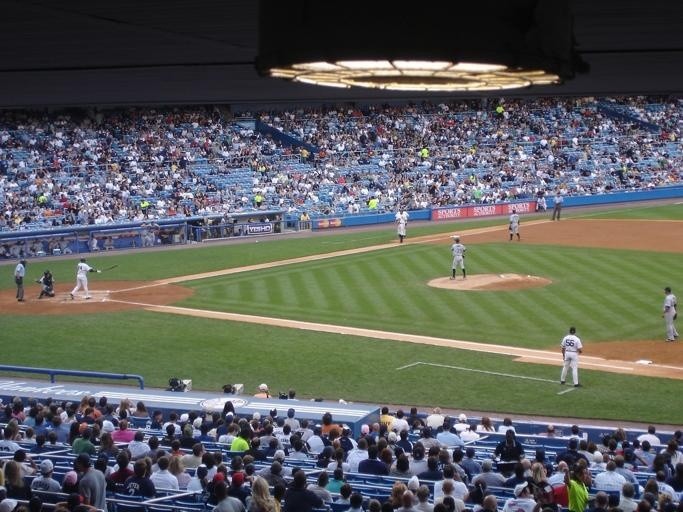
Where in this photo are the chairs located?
[0,103,683,239]
[1,412,683,511]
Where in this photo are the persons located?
[35,270,55,299]
[450,238,467,280]
[14,259,26,302]
[663,287,679,342]
[561,327,583,387]
[0,91,683,260]
[70,256,101,300]
[0,377,683,512]
